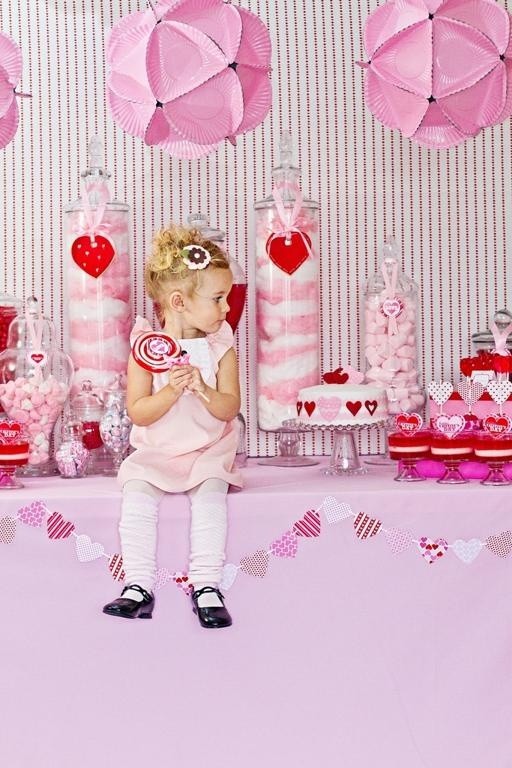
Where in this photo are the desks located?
[0,452,512,764]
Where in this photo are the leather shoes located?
[192,587,232,628]
[103,584,155,618]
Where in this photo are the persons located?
[100,220,244,629]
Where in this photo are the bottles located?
[64,135,132,384]
[252,129,326,468]
[364,237,427,467]
[472,309,512,389]
[152,214,247,339]
[98,390,132,473]
[70,378,103,451]
[1,296,73,478]
[57,412,89,478]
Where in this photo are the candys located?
[250,194,512,473]
[133,328,213,405]
[0,191,133,474]
[153,248,247,337]
